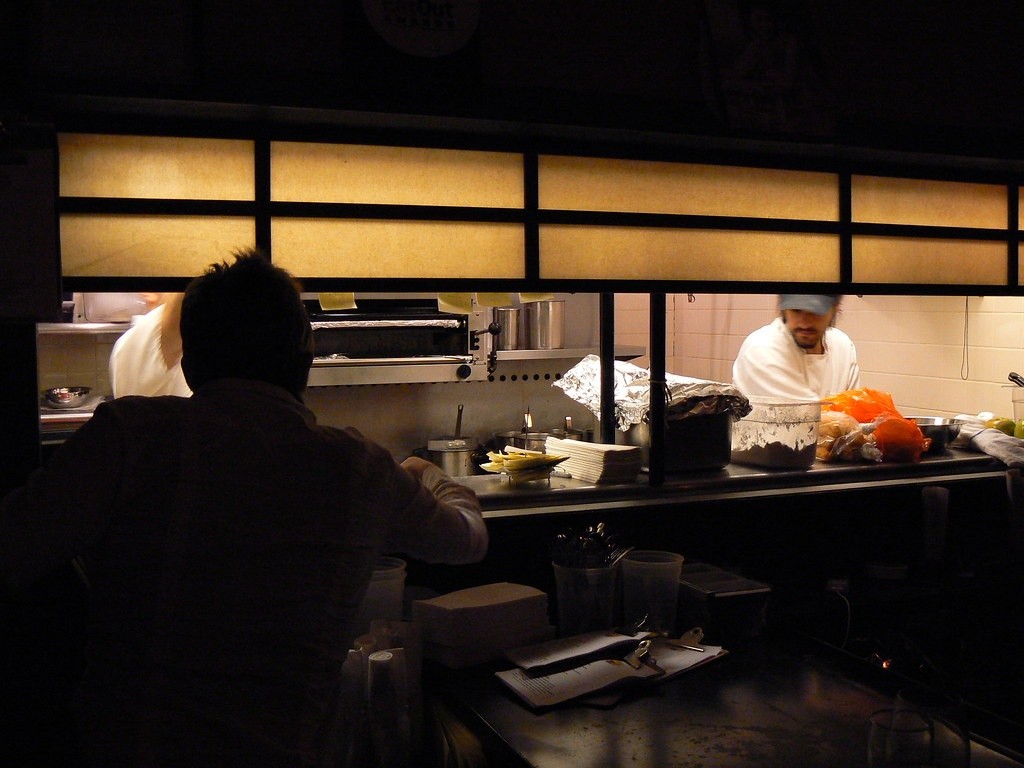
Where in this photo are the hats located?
[776,293,838,315]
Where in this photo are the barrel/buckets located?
[590,402,732,474]
[493,307,521,351]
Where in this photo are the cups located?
[1010,388,1023,422]
[621,550,683,635]
[551,560,620,637]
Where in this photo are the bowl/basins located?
[40,386,91,409]
[906,416,965,449]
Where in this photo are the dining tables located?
[450,588,1024,768]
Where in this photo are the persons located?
[1,244,492,768]
[109,293,193,399]
[731,295,862,399]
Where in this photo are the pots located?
[525,298,565,351]
[429,436,479,477]
[493,430,594,455]
